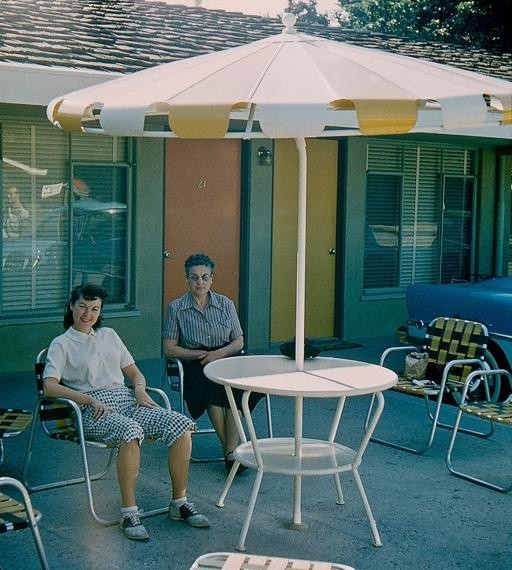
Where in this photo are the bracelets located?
[134,383,145,387]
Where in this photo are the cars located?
[57,198,127,280]
[401,275,511,408]
[2,213,64,284]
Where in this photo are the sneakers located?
[169,501,211,528]
[119,508,150,541]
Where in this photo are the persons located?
[42,283,211,540]
[161,253,266,477]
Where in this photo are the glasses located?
[186,273,212,281]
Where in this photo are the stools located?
[1,474,57,569]
[446,367,511,497]
[189,548,359,570]
[1,402,33,463]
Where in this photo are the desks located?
[202,352,401,553]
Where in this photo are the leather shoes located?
[225,451,244,474]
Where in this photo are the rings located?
[99,409,103,413]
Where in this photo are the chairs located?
[156,348,251,465]
[369,315,498,476]
[24,345,194,527]
